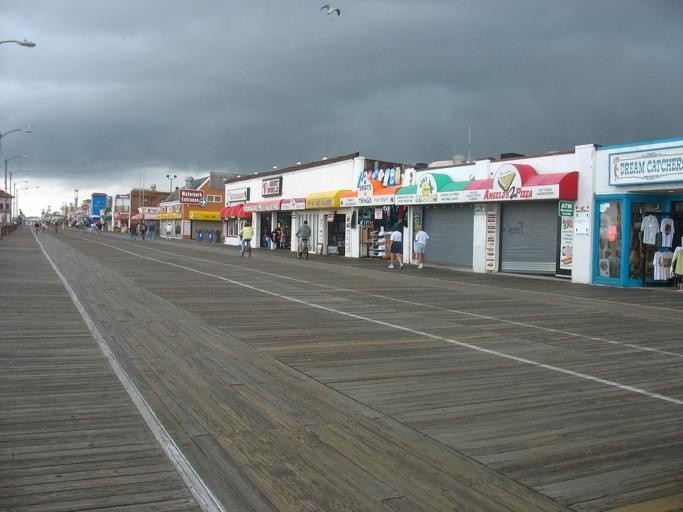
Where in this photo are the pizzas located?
[498,172,516,192]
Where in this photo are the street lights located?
[0,38,38,49]
[1,126,41,225]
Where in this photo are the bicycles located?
[296,235,309,260]
[239,234,253,259]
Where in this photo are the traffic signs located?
[179,189,207,207]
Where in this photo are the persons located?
[295,219,311,252]
[387,225,404,269]
[34,218,103,235]
[129,221,181,241]
[239,221,253,253]
[413,225,430,269]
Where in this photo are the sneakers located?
[389,264,394,268]
[400,265,404,268]
[417,264,424,268]
